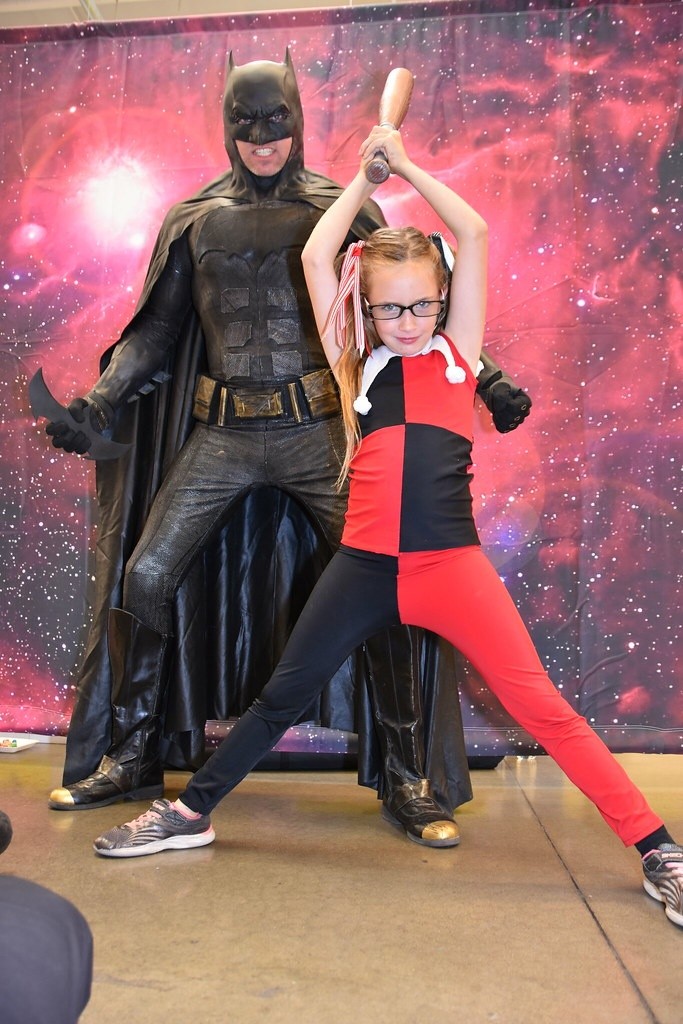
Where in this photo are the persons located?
[47,60,534,847]
[93,125,680,931]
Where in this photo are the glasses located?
[364,291,447,320]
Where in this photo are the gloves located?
[45,395,108,456]
[481,372,532,435]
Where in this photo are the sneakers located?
[639,841,683,929]
[91,798,216,858]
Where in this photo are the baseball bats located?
[365,68,415,183]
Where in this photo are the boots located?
[47,607,170,812]
[364,620,462,849]
